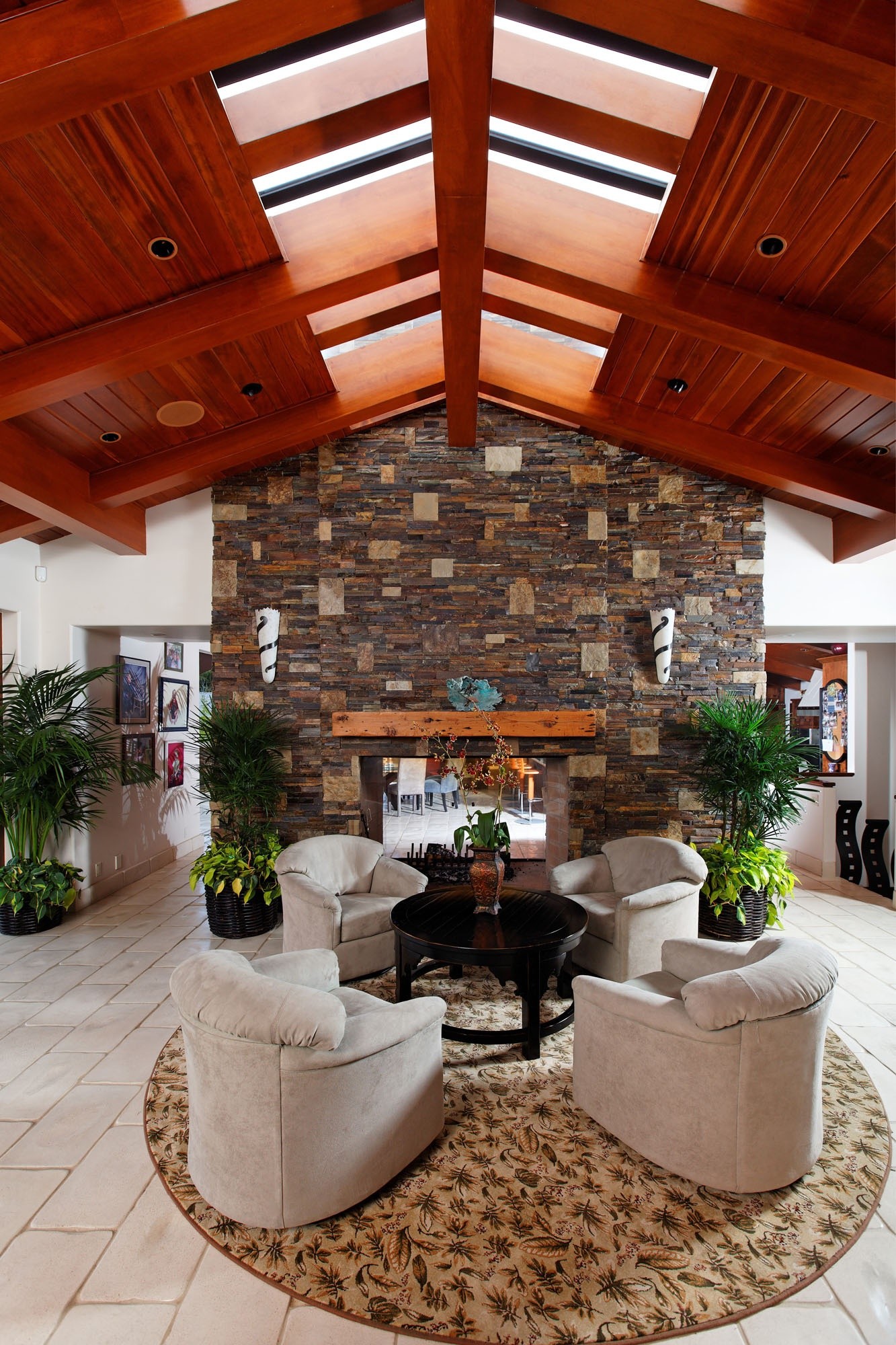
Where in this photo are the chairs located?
[416,758,464,812]
[387,758,427,817]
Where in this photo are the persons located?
[822,689,847,746]
[171,750,181,782]
[134,742,144,763]
[123,673,134,715]
[166,649,181,669]
[169,689,179,724]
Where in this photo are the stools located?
[514,769,546,824]
[501,761,527,801]
[506,761,534,807]
[507,763,537,814]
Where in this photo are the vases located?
[466,843,505,915]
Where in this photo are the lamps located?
[649,608,675,684]
[254,608,280,684]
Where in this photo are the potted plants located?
[0,650,163,936]
[180,690,294,940]
[673,687,825,942]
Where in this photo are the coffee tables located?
[389,885,590,1061]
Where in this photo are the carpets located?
[142,955,893,1345]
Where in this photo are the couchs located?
[169,947,447,1230]
[571,934,841,1194]
[274,834,429,982]
[549,836,708,984]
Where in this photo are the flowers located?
[406,685,521,854]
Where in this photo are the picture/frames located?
[164,739,186,791]
[116,655,151,725]
[121,733,155,786]
[164,641,184,673]
[158,677,190,732]
